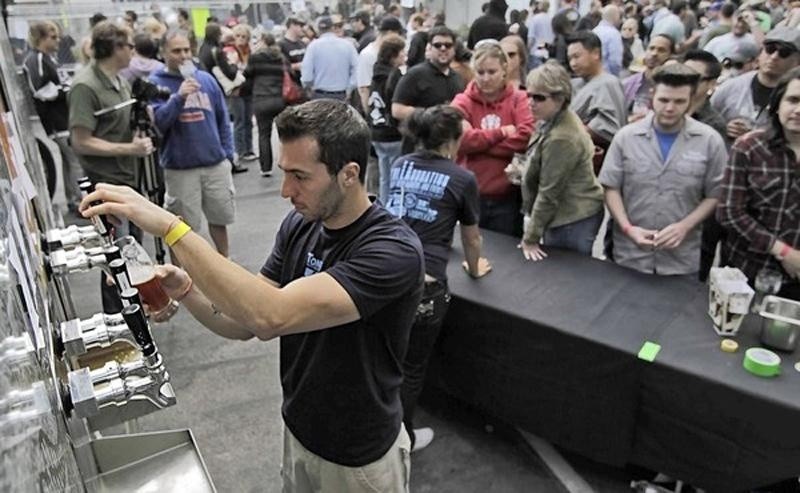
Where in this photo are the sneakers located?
[412,427,434,452]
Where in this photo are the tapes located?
[720,338,739,353]
[742,347,782,377]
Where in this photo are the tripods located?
[129,109,166,264]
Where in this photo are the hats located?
[318,18,331,29]
[382,19,401,30]
[762,27,800,49]
[349,10,369,24]
[286,16,305,24]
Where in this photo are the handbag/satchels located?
[282,57,302,103]
[212,65,246,96]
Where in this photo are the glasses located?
[723,58,743,69]
[527,93,551,101]
[432,43,453,48]
[766,42,792,57]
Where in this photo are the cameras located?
[130,76,171,103]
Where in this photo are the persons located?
[83,3,799,237]
[598,64,730,281]
[714,65,800,294]
[507,61,604,262]
[70,16,156,253]
[385,105,491,455]
[145,30,235,260]
[77,98,426,493]
[25,20,83,215]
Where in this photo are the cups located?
[178,58,200,85]
[106,235,180,322]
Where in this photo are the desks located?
[421,222,706,482]
[628,280,800,492]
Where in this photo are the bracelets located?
[174,277,194,302]
[621,222,637,238]
[773,243,794,266]
[158,217,192,246]
